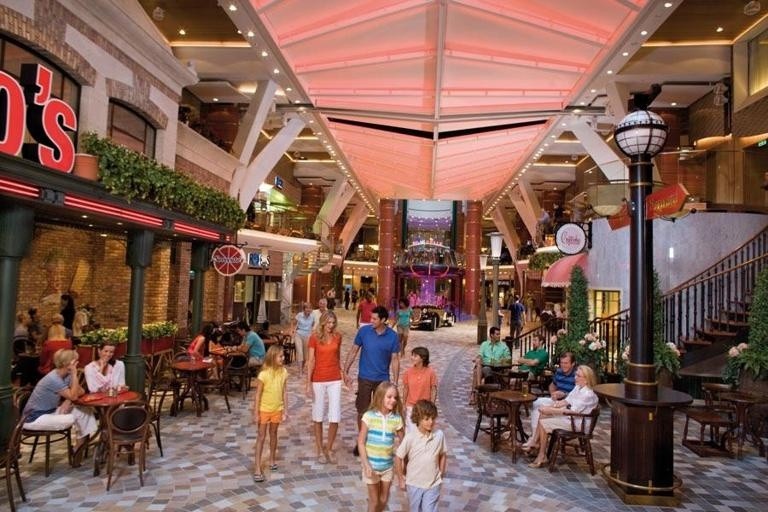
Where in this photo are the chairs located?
[118,387,168,466]
[101,400,152,492]
[468,348,768,477]
[2,410,32,512]
[147,332,295,414]
[14,387,73,477]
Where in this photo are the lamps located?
[151,6,165,24]
[741,1,761,17]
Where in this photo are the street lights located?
[477,252,490,347]
[484,231,506,344]
[256,252,271,325]
[593,109,694,496]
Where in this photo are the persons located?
[537,206,550,223]
[344,306,400,456]
[520,365,599,468]
[506,295,527,349]
[356,293,375,327]
[291,299,328,372]
[541,302,566,320]
[393,298,414,345]
[21,348,100,468]
[469,327,510,404]
[393,399,447,511]
[418,308,455,326]
[84,338,128,393]
[188,320,266,368]
[327,290,359,310]
[523,351,577,445]
[305,312,348,462]
[552,203,564,220]
[254,344,290,480]
[505,335,549,390]
[402,347,438,419]
[14,294,92,353]
[359,381,405,511]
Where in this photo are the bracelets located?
[339,367,345,371]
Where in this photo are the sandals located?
[529,458,548,468]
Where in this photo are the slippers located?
[269,464,277,470]
[254,473,264,482]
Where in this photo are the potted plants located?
[71,131,130,194]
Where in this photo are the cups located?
[521,381,529,396]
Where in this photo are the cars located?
[405,303,457,331]
